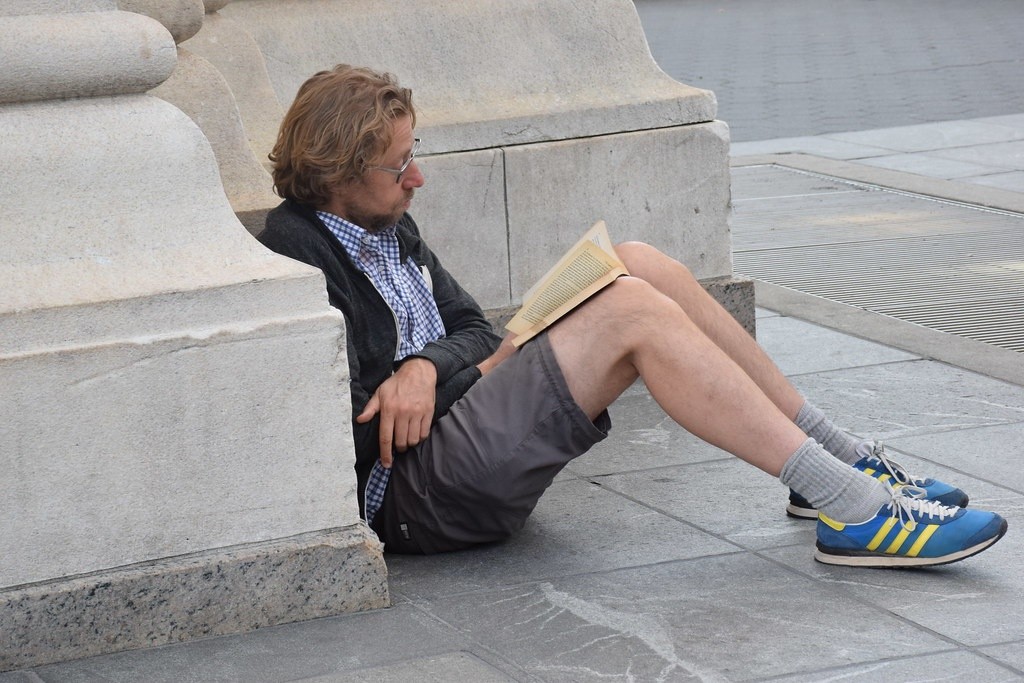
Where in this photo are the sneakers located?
[813,478,1008,568]
[785,439,970,521]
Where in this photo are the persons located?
[255,63,1008,569]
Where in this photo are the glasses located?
[363,137,422,184]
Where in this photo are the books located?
[505,219,629,348]
